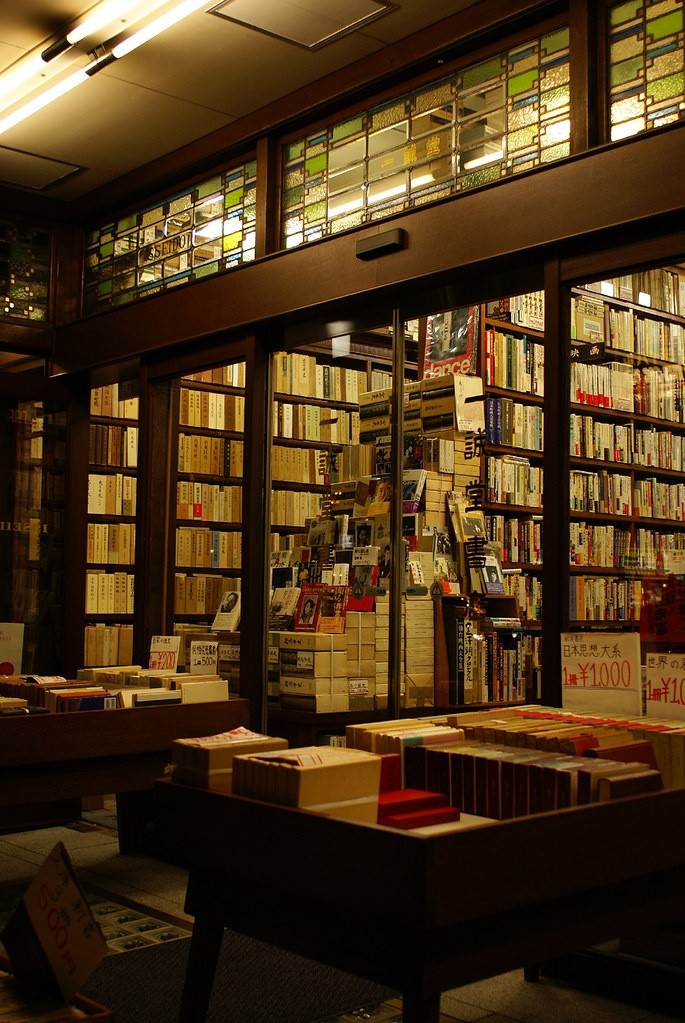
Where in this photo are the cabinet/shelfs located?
[0,258,684,1023]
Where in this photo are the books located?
[83,574,243,665]
[484,456,685,621]
[11,410,45,624]
[483,268,684,472]
[90,350,417,486]
[443,617,543,705]
[171,704,684,836]
[185,546,434,714]
[88,474,325,569]
[304,372,484,552]
[0,664,229,716]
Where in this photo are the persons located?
[220,592,239,613]
[377,545,391,584]
[436,565,447,578]
[357,528,369,546]
[449,569,458,583]
[298,563,309,587]
[298,600,314,625]
[370,479,391,502]
[490,571,500,582]
